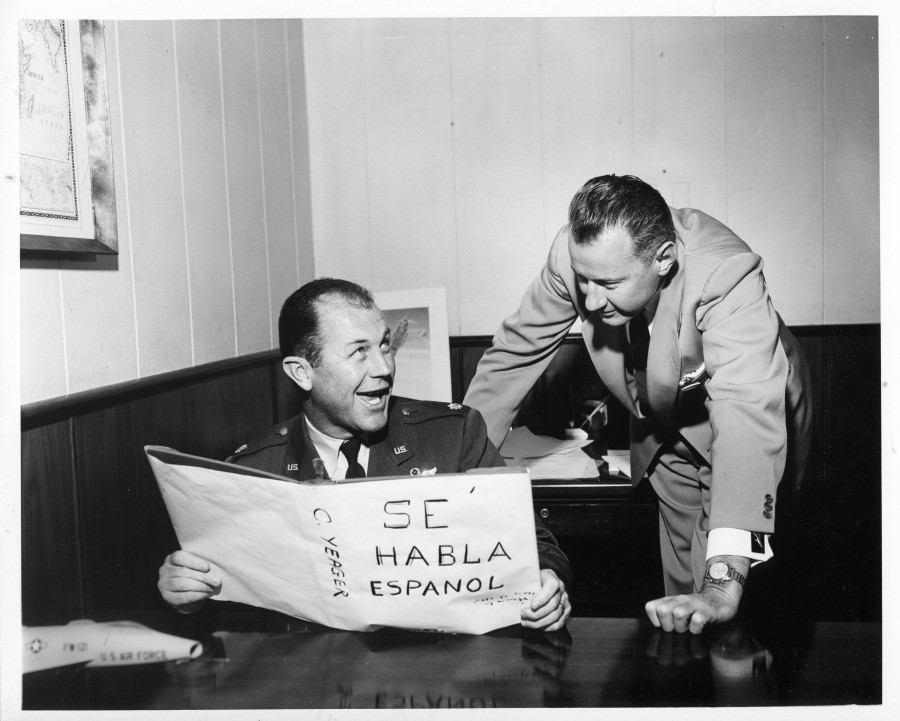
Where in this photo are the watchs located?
[704,561,746,588]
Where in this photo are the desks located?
[22,611,882,711]
[532,462,666,618]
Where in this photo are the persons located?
[166,633,573,693]
[649,628,779,707]
[158,280,572,635]
[461,175,810,633]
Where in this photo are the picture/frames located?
[19,18,121,256]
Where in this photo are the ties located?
[631,311,656,416]
[340,437,366,479]
